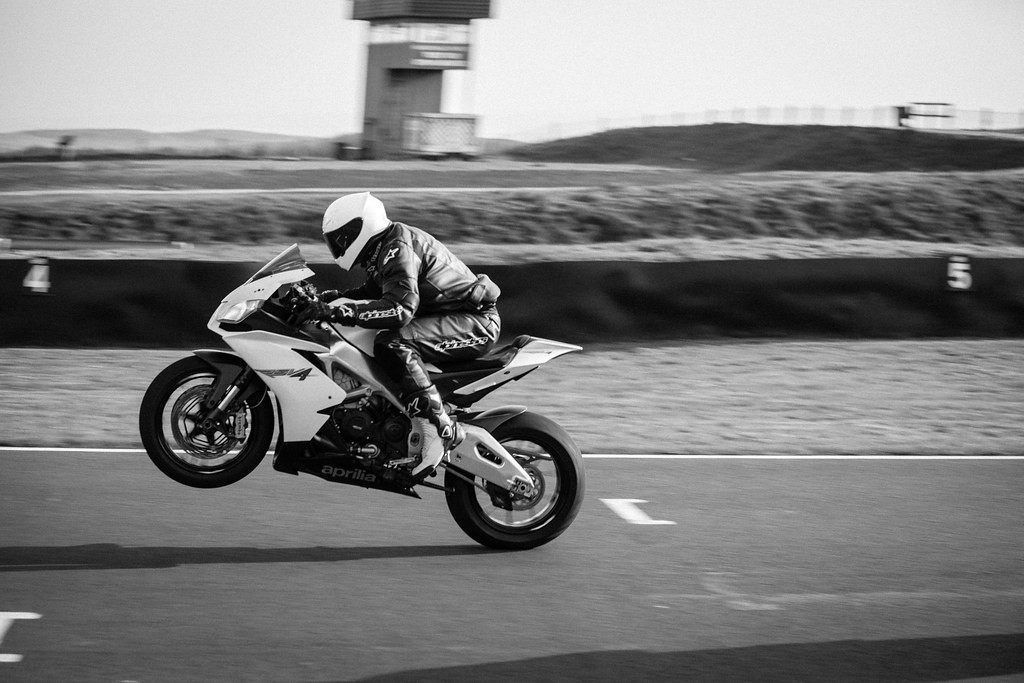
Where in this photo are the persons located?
[300,192,501,482]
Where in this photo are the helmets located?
[322,191,391,272]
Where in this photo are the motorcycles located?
[138,242,590,552]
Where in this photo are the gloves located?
[292,296,331,323]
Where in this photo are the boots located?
[401,383,467,479]
